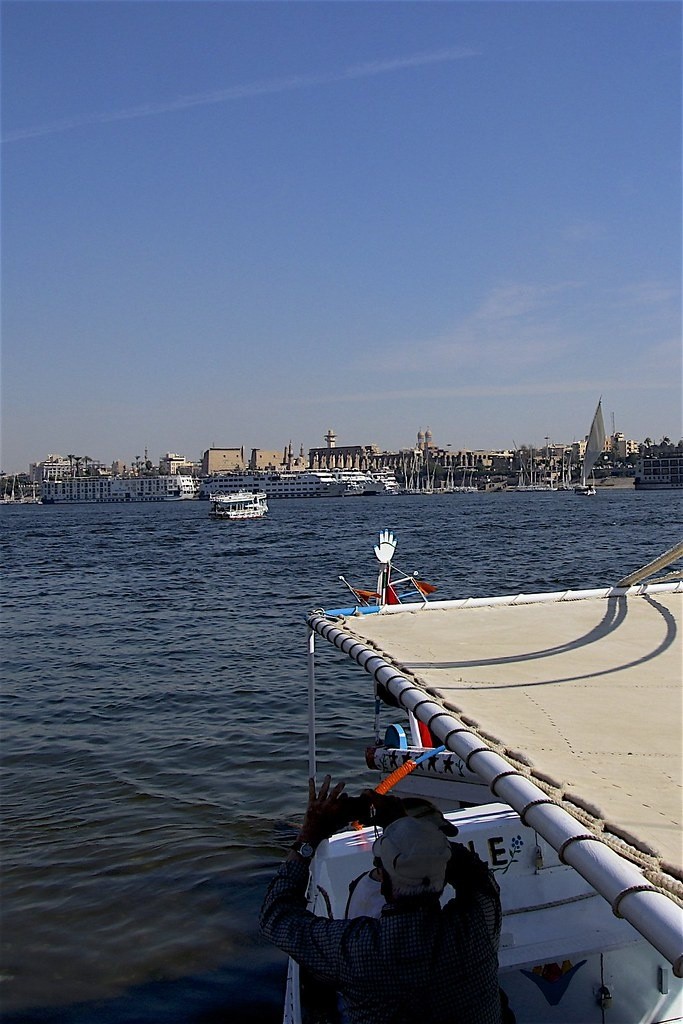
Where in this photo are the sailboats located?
[0,477,38,503]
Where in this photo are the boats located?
[208,488,269,520]
[576,489,596,496]
[381,486,478,496]
[284,543,683,1024]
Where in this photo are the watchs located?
[291,842,315,858]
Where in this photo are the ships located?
[40,474,195,502]
[200,467,396,499]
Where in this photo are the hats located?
[372,816,453,892]
[383,798,459,837]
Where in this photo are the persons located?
[258,774,502,1024]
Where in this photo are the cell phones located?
[336,796,372,821]
